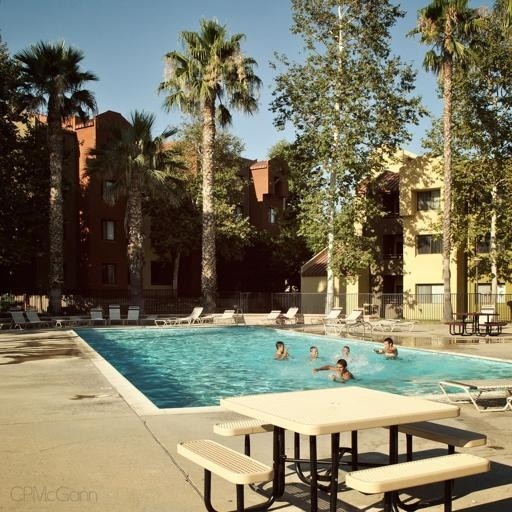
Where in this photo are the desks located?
[452,312,500,337]
[220,385,460,512]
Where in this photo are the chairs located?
[438,377,511,413]
[262,307,299,326]
[6,303,235,331]
[310,306,419,335]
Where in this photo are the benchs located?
[444,321,507,336]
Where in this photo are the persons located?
[373,337,398,361]
[309,346,319,362]
[274,341,289,361]
[331,345,352,365]
[311,359,353,384]
